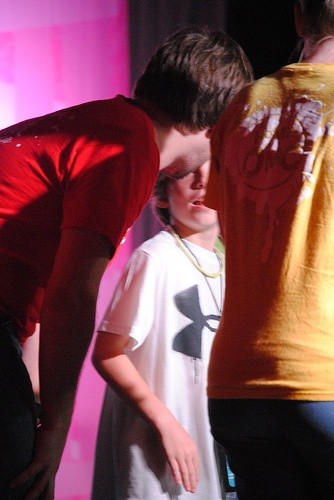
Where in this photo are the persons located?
[0,23,254,500]
[93,132,229,500]
[203,0,334,499]
